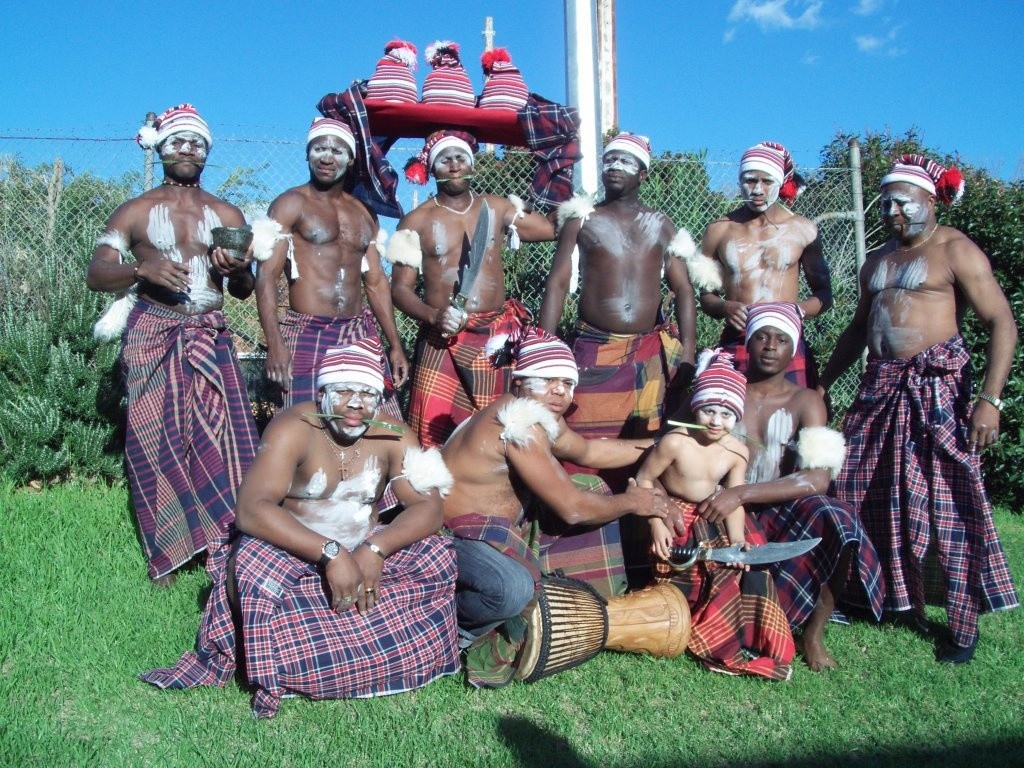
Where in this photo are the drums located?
[516,571,694,678]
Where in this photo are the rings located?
[176,271,180,278]
[365,587,374,595]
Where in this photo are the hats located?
[690,347,748,422]
[488,326,579,387]
[744,300,806,359]
[602,131,651,173]
[422,39,477,108]
[738,141,797,199]
[317,335,393,401]
[364,38,420,104]
[306,116,357,160]
[135,103,213,152]
[880,153,966,206]
[402,130,480,187]
[478,47,529,111]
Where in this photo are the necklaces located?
[432,189,474,214]
[896,222,939,252]
[317,404,362,481]
[161,180,202,187]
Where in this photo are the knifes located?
[441,195,489,338]
[646,537,825,567]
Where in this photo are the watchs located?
[318,541,341,570]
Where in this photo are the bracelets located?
[977,392,1005,412]
[364,539,387,560]
[653,435,661,447]
[133,259,144,281]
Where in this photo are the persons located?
[821,155,1021,663]
[538,133,722,490]
[386,129,570,450]
[85,104,258,588]
[699,141,834,424]
[436,326,670,654]
[636,346,823,681]
[653,301,886,671]
[135,337,462,719]
[255,116,409,516]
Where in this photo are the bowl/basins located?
[209,224,253,260]
